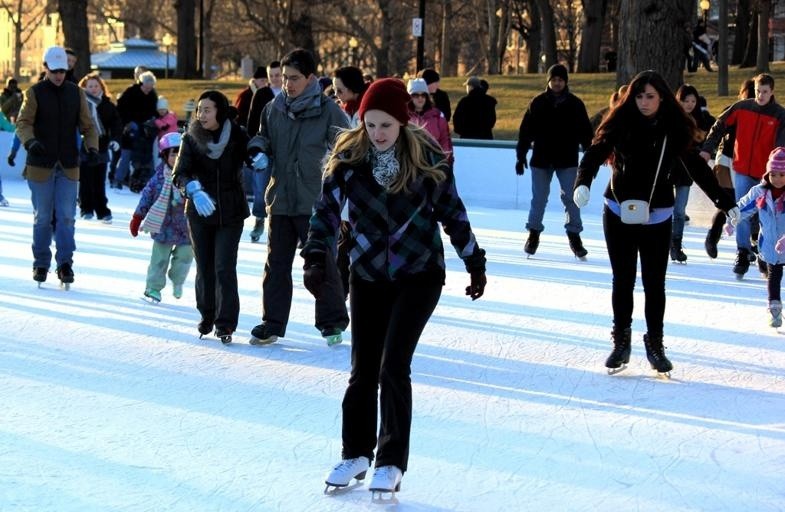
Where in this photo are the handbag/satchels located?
[620,198,651,225]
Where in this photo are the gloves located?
[186,180,215,218]
[85,150,97,166]
[302,254,328,300]
[516,157,528,176]
[252,152,269,174]
[130,214,144,236]
[572,185,591,208]
[464,269,487,301]
[724,222,735,235]
[107,139,121,154]
[774,234,785,255]
[728,206,743,225]
[23,140,46,158]
[248,148,262,169]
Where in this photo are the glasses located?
[411,90,428,99]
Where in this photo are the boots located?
[642,333,674,379]
[670,235,688,265]
[566,231,589,261]
[523,228,542,259]
[756,254,770,281]
[248,216,265,243]
[604,326,631,375]
[768,300,783,328]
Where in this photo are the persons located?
[15,46,102,282]
[0,79,23,121]
[129,131,195,305]
[571,69,741,380]
[691,18,716,71]
[172,89,270,343]
[8,71,76,250]
[65,47,85,83]
[681,22,695,71]
[300,78,487,501]
[599,47,617,72]
[77,66,178,221]
[318,66,374,299]
[406,68,497,170]
[669,74,785,328]
[232,61,281,240]
[246,49,354,346]
[515,64,600,257]
[587,84,628,131]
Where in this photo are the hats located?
[358,79,409,128]
[421,69,438,85]
[546,65,569,85]
[43,47,71,72]
[155,95,168,111]
[766,147,785,173]
[407,77,430,102]
[463,78,481,89]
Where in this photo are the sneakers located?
[369,463,405,505]
[322,454,370,496]
[56,262,74,293]
[732,246,752,282]
[248,319,285,346]
[705,230,719,259]
[0,197,10,208]
[138,283,161,307]
[215,320,234,342]
[196,318,215,340]
[82,212,94,221]
[31,266,49,287]
[316,324,345,347]
[170,274,184,299]
[100,215,116,224]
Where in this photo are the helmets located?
[158,132,187,155]
